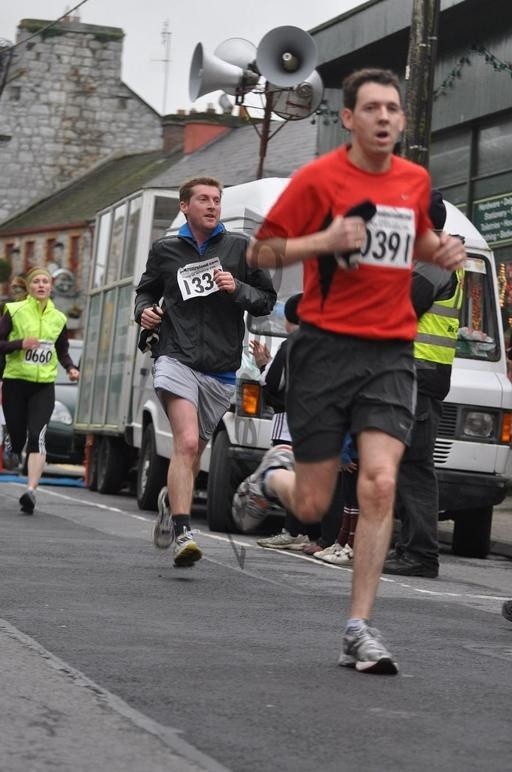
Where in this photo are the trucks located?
[73,177,512,559]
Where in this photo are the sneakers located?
[337,621,399,674]
[256,527,324,555]
[312,541,344,559]
[19,490,36,516]
[172,532,203,567]
[231,443,296,532]
[381,550,439,579]
[322,543,353,566]
[153,485,176,550]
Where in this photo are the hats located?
[427,190,447,232]
[284,292,304,324]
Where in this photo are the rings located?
[354,240,361,249]
[354,224,360,232]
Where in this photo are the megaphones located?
[255,25,317,87]
[264,69,324,121]
[187,41,244,105]
[214,36,260,97]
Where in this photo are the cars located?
[46,340,96,465]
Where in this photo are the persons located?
[1,266,81,516]
[245,69,470,676]
[371,185,463,576]
[303,477,341,555]
[132,177,277,568]
[249,291,310,552]
[310,433,363,564]
